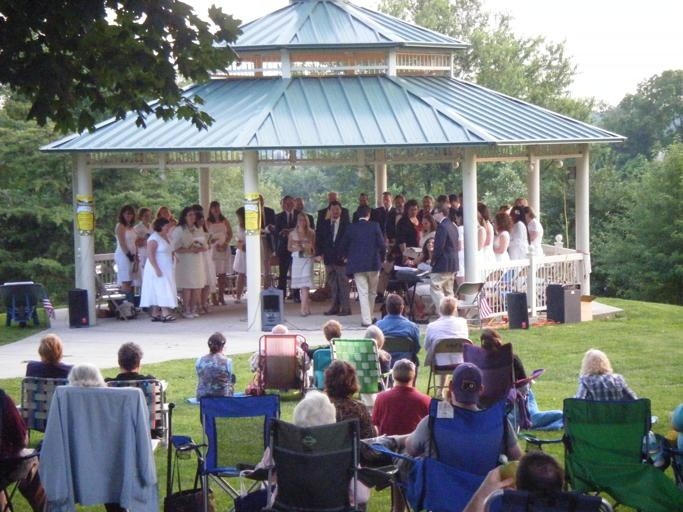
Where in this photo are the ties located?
[330,219,336,242]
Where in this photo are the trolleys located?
[0,282,40,328]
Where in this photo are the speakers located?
[546,283,583,322]
[69,288,88,327]
[507,293,528,328]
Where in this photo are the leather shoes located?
[337,308,352,315]
[324,306,339,315]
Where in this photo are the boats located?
[103,273,278,301]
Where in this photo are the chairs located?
[194,395,682,511]
[228,271,237,299]
[378,260,420,324]
[453,282,486,326]
[260,335,515,395]
[270,255,280,288]
[313,255,324,289]
[0,378,174,511]
[95,276,129,321]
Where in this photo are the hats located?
[451,362,484,405]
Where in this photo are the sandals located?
[153,298,242,320]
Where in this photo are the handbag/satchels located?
[162,486,217,512]
